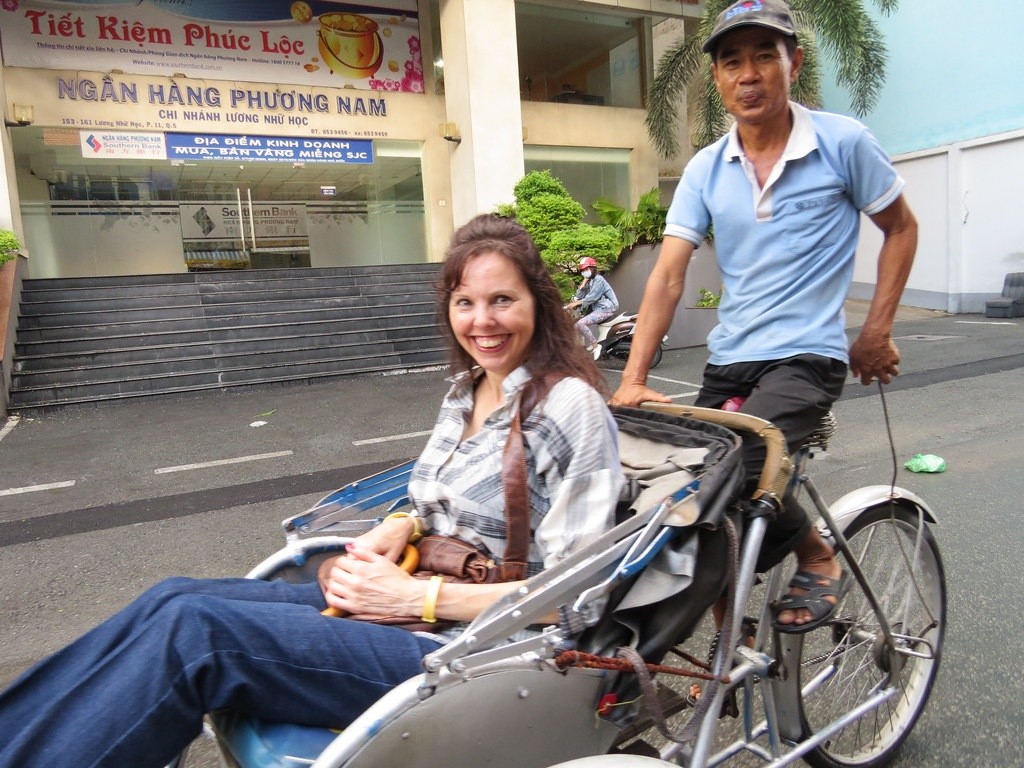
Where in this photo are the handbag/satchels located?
[318,535,526,632]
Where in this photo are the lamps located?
[4,103,35,127]
[522,126,529,142]
[438,123,461,144]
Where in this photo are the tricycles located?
[197,335,949,768]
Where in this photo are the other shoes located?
[585,345,593,351]
[593,344,602,360]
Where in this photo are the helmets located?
[578,257,598,270]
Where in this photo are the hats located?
[702,0,797,54]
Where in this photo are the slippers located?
[768,565,855,634]
[686,664,708,708]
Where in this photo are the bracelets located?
[420,575,443,624]
[382,510,423,542]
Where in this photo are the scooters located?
[562,303,671,369]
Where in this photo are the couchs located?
[548,92,604,106]
[985,272,1024,318]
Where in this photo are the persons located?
[566,257,619,360]
[0,213,630,768]
[607,0,918,710]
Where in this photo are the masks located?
[581,271,591,278]
